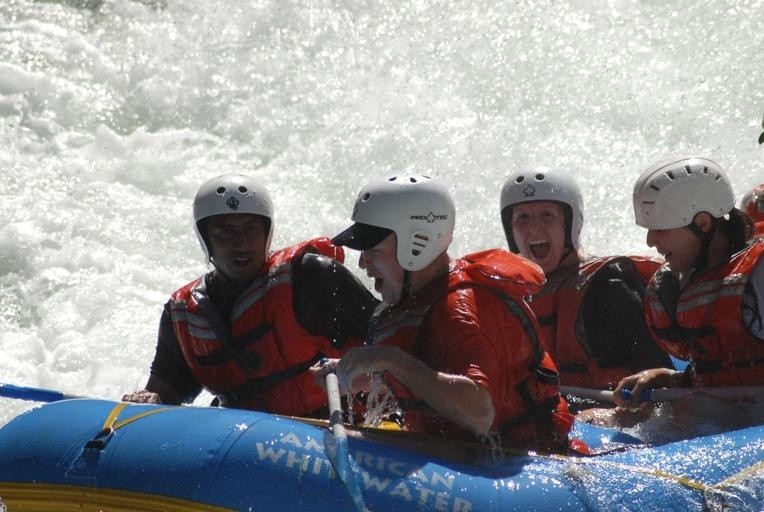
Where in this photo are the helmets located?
[194,173,275,264]
[633,153,736,231]
[351,173,456,271]
[499,166,585,254]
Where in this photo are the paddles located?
[568,422,645,457]
[319,356,369,512]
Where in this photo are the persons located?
[498,170,676,416]
[572,151,764,445]
[328,171,591,458]
[120,171,380,428]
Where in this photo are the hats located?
[332,223,393,251]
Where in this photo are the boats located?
[0,396,764,512]
